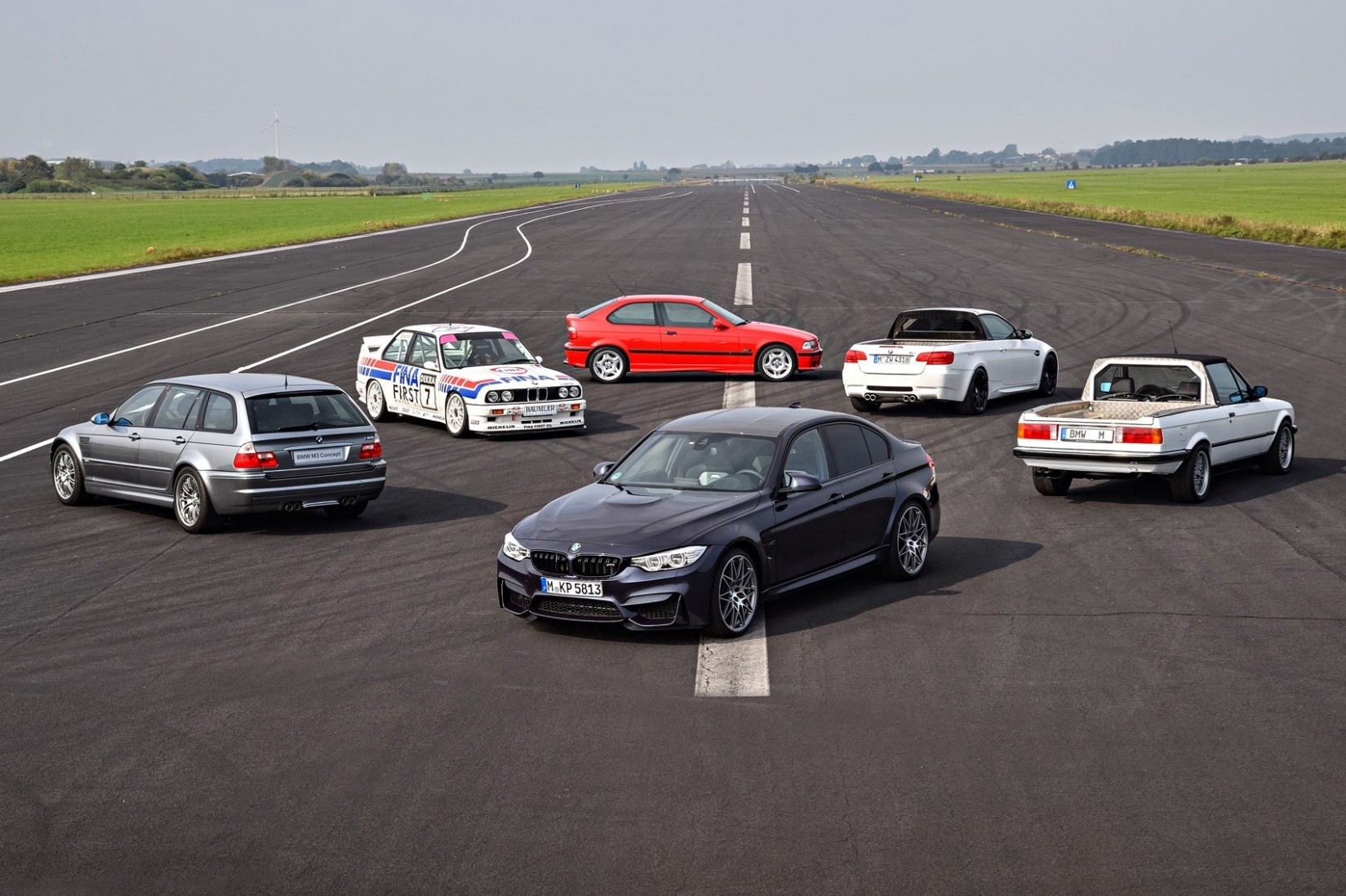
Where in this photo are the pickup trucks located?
[1011,354,1299,504]
[842,307,1059,415]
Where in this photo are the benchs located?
[255,403,313,432]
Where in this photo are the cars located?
[496,401,940,640]
[50,373,388,535]
[563,294,824,385]
[356,323,587,439]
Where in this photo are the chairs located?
[467,341,498,366]
[686,441,735,480]
[759,442,815,478]
[1113,377,1135,399]
[1176,382,1201,401]
[177,396,201,429]
